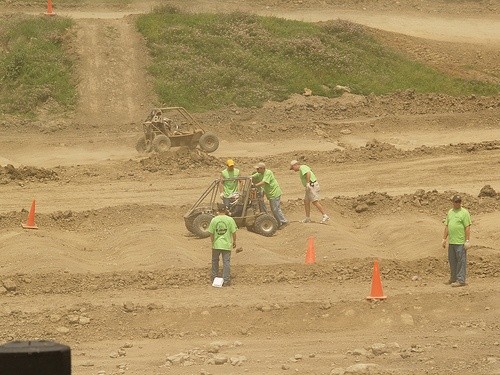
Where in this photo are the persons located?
[442,195,472,287]
[289,160,329,224]
[218,159,243,215]
[251,163,287,228]
[207,204,239,286]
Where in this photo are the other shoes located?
[299,217,311,223]
[222,280,230,286]
[277,220,288,230]
[320,217,330,224]
[451,282,466,286]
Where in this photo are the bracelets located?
[234,241,236,243]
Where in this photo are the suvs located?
[183,176,278,238]
[135,106,219,155]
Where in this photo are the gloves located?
[464,242,470,249]
[220,193,224,199]
[442,239,446,248]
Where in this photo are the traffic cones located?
[21,199,38,229]
[365,259,388,299]
[302,238,316,264]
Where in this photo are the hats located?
[227,159,234,166]
[453,195,461,202]
[289,160,298,170]
[254,163,265,169]
